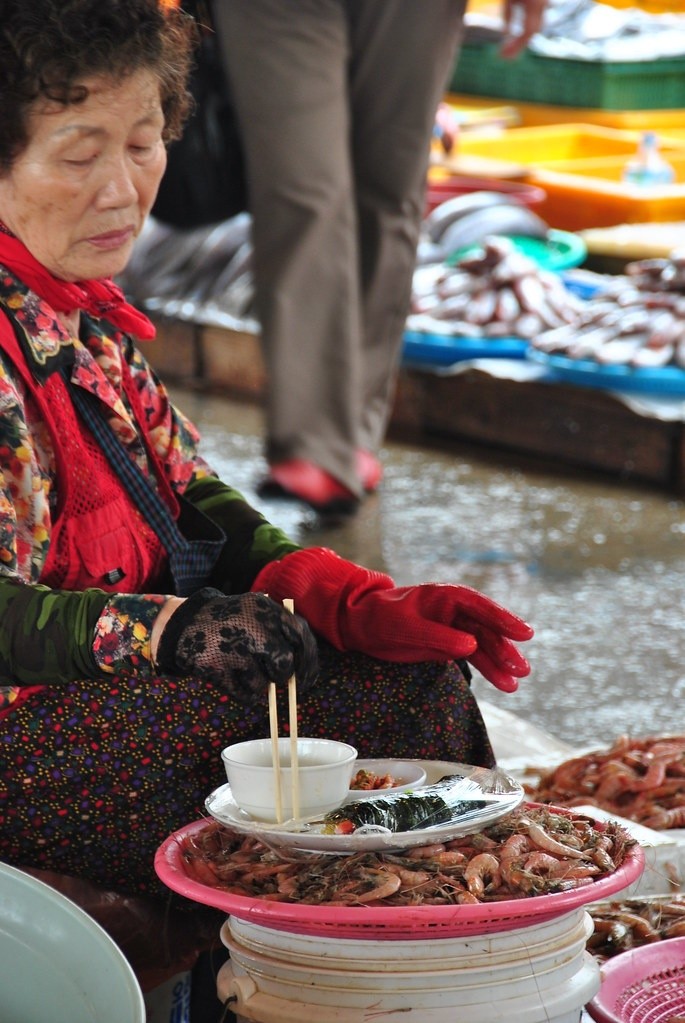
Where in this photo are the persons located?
[0,1,533,1023]
[159,0,546,513]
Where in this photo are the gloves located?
[252,546,534,694]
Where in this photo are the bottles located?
[624,132,674,185]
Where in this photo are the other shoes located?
[256,459,357,517]
[353,449,381,493]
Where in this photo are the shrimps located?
[173,726,685,964]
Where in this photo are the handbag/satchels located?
[141,0,252,233]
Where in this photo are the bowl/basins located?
[450,227,588,275]
[221,737,358,822]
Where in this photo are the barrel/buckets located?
[215,915,603,1023]
[0,859,149,1023]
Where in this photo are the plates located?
[344,759,427,802]
[204,757,527,855]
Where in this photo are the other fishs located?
[111,209,685,371]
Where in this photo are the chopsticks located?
[260,593,301,823]
